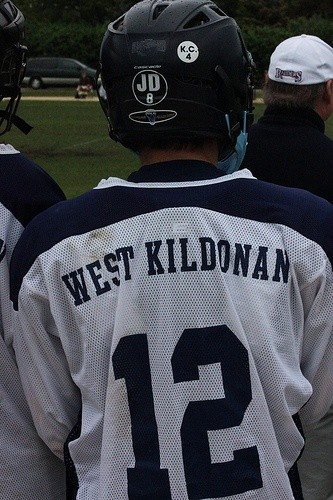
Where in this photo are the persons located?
[79,71,93,93]
[239,34,333,204]
[8,0,333,500]
[0,0,66,500]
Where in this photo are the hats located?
[268,34,333,86]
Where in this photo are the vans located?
[19,57,97,90]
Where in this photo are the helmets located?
[0,0,31,96]
[92,0,258,139]
